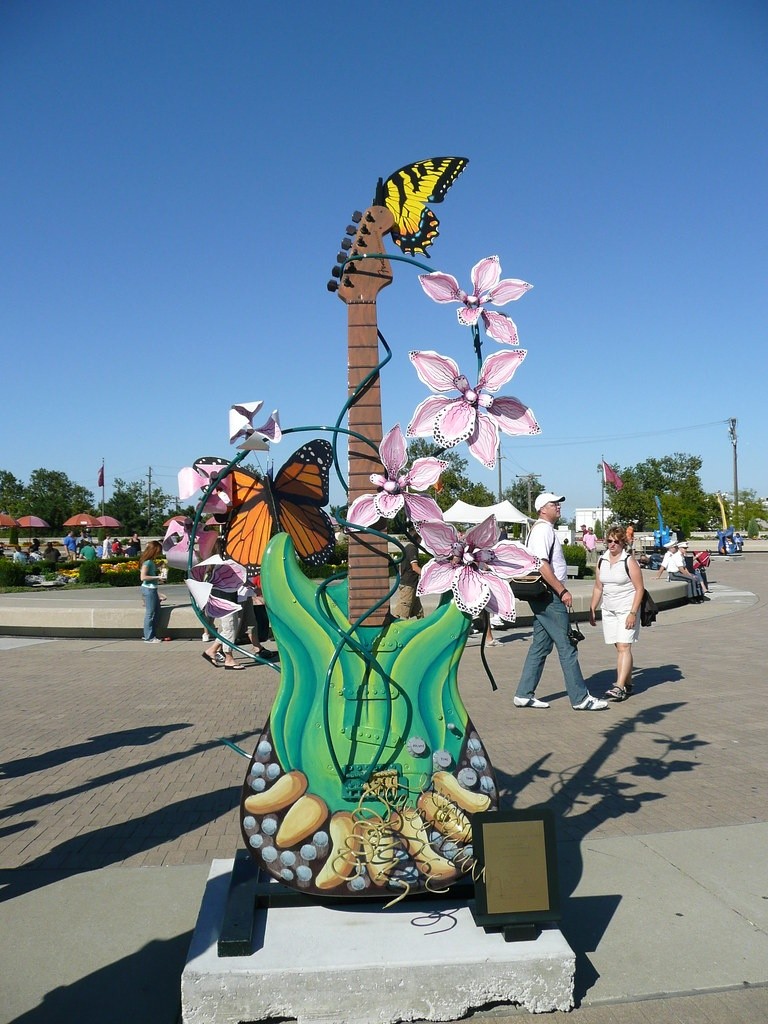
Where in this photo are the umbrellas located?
[163,516,194,526]
[17,516,49,546]
[0,512,22,538]
[63,513,123,539]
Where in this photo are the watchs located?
[630,612,637,618]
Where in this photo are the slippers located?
[201,651,224,669]
[224,664,245,670]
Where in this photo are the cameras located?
[568,630,585,646]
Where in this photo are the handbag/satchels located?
[509,571,552,601]
[640,589,658,627]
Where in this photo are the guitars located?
[654,495,669,547]
[717,494,737,554]
[240,204,499,903]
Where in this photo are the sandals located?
[604,684,637,703]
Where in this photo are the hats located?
[677,542,688,548]
[533,491,566,511]
[663,541,676,548]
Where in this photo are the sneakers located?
[512,695,549,709]
[571,694,609,712]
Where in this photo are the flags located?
[603,461,624,492]
[98,464,105,487]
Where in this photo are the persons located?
[512,492,609,711]
[499,526,509,542]
[0,538,61,563]
[63,530,141,561]
[578,525,597,564]
[653,540,710,604]
[457,525,473,541]
[140,540,167,643]
[202,537,274,671]
[394,532,422,618]
[589,526,643,700]
[725,533,743,555]
[626,522,635,554]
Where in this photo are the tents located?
[443,499,538,537]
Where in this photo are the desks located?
[3,543,64,553]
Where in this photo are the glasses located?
[607,539,622,544]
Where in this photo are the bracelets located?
[250,585,258,590]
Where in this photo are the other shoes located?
[254,647,277,663]
[689,597,705,603]
[144,637,162,643]
